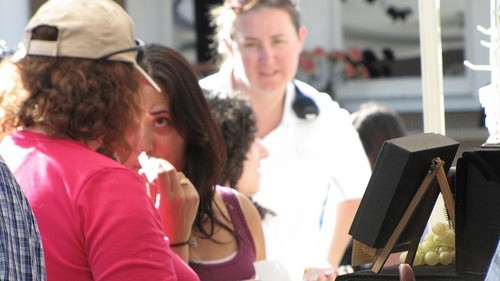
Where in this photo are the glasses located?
[94,38,145,64]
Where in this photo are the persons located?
[201,89,276,221]
[0,153,50,280]
[0,0,203,280]
[109,41,271,280]
[188,0,375,281]
[346,102,406,177]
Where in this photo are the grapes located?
[400,222,455,265]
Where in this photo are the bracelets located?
[168,236,198,248]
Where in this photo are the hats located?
[18,0,162,93]
